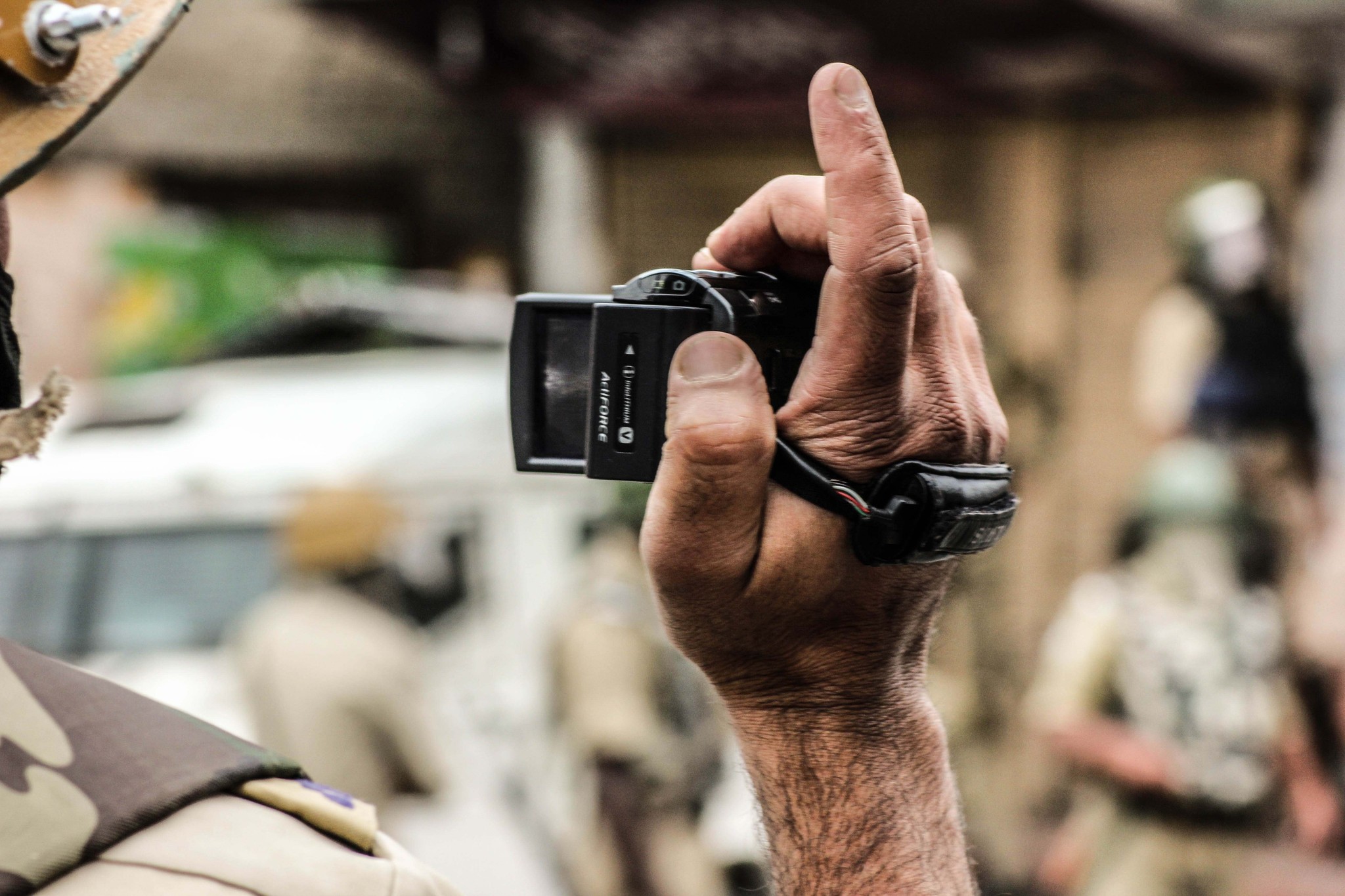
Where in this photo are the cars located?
[0,344,790,896]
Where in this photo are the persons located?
[2,0,1018,896]
[1008,434,1345,896]
[1119,168,1318,703]
[527,504,738,896]
[205,461,453,844]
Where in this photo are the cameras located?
[507,265,816,482]
